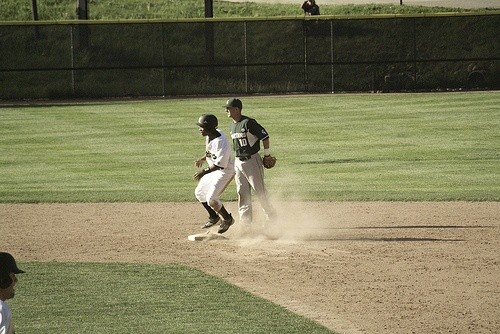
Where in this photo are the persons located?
[221,98,282,240]
[194,114,236,233]
[0,252,26,334]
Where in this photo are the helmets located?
[0,252,26,288]
[195,113,219,134]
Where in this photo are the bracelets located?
[205,167,211,173]
[264,148,270,156]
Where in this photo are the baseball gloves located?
[262,154,276,169]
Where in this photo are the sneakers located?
[201,215,220,229]
[217,213,235,233]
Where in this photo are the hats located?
[221,98,243,110]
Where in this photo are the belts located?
[239,155,252,161]
